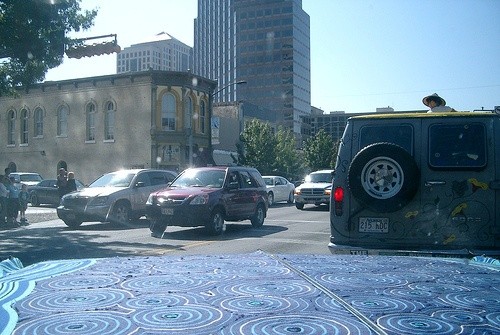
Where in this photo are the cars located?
[25,178,86,207]
[261,175,296,207]
[57,168,179,230]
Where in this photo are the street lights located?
[208,79,247,165]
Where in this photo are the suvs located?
[145,166,269,237]
[294,169,335,210]
[328,112,500,259]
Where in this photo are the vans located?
[9,172,44,192]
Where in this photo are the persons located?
[422,93,456,113]
[57,168,77,199]
[0,167,30,227]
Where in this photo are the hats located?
[422,93,446,107]
